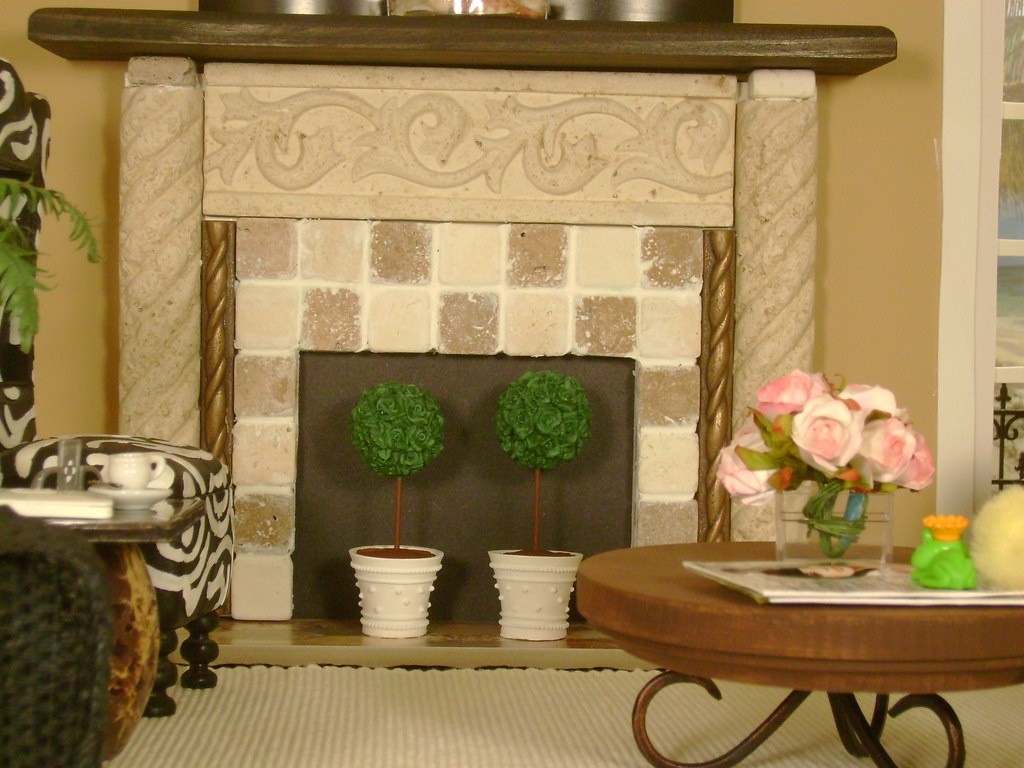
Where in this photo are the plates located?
[89,487,171,512]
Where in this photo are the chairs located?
[1,59,234,720]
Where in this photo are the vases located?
[773,482,892,574]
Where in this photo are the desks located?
[0,493,206,762]
[575,542,1024,768]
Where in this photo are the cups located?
[106,450,165,488]
[58,436,81,490]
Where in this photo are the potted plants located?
[487,372,592,640]
[348,380,445,639]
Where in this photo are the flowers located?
[714,370,936,562]
